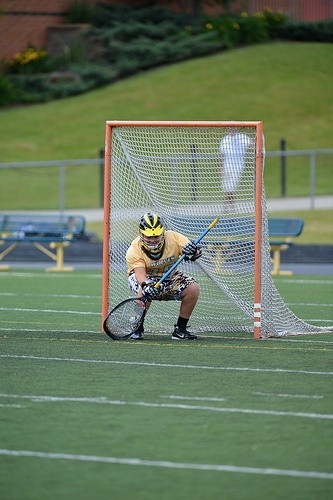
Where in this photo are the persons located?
[219,126,253,214]
[126,213,201,341]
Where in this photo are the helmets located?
[139,212,164,254]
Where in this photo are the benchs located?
[162,216,304,277]
[0,213,85,272]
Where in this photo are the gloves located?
[182,242,197,261]
[140,279,163,302]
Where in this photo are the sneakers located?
[130,325,143,339]
[171,324,197,340]
